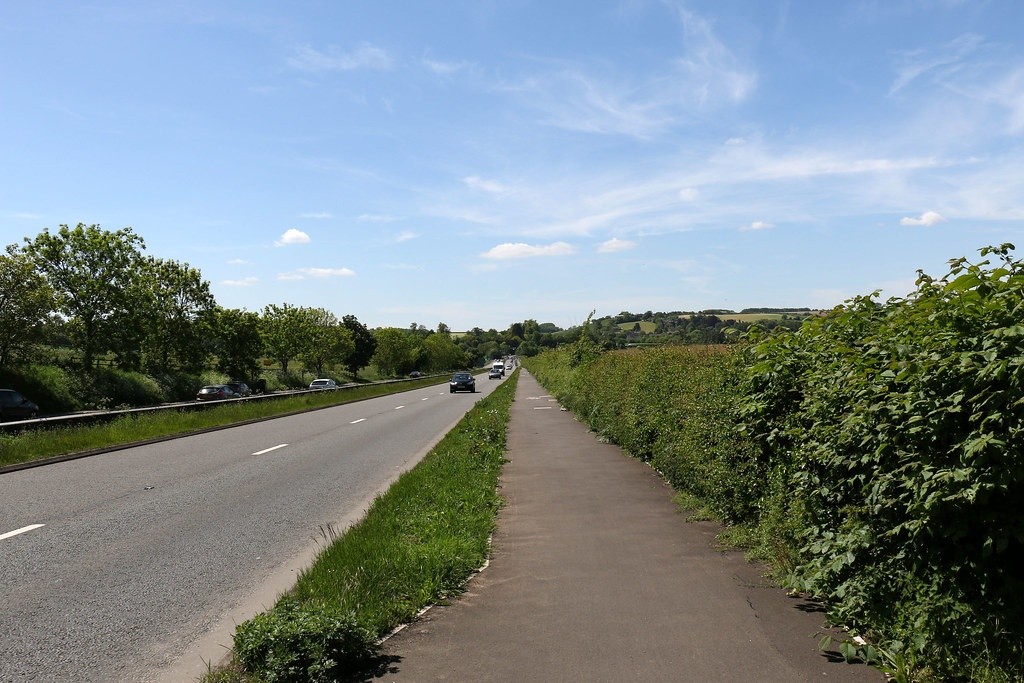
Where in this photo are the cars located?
[505,361,514,370]
[0,388,40,422]
[227,380,253,397]
[195,384,241,402]
[409,371,421,379]
[449,372,477,393]
[488,369,501,379]
[308,378,339,391]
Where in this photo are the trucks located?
[492,360,506,377]
